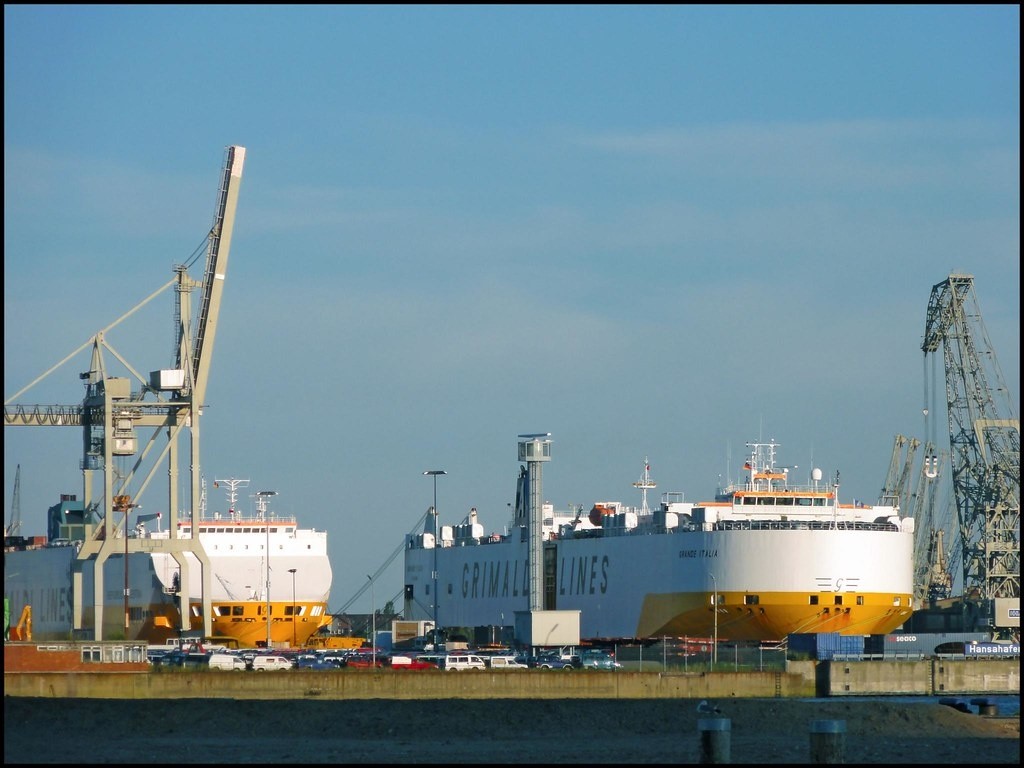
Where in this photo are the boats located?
[8,463,332,646]
[403,412,913,640]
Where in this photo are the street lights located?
[288,569,297,647]
[709,573,717,665]
[368,574,376,664]
[422,471,448,651]
[257,491,277,645]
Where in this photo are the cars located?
[147,637,714,670]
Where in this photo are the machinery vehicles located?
[16,606,32,641]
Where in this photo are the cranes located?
[6,145,245,641]
[921,273,1022,643]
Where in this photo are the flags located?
[743,463,752,470]
